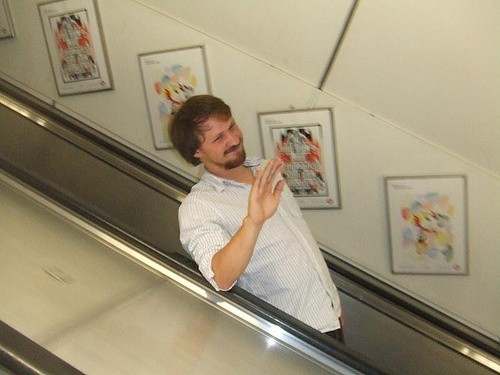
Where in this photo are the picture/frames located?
[384,173,470,277]
[257,106,343,211]
[36,0,116,97]
[137,44,214,151]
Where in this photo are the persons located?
[167,94,346,344]
